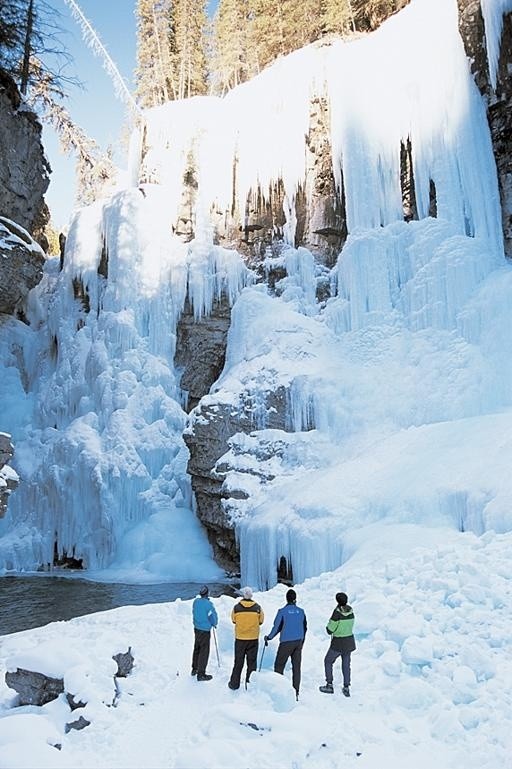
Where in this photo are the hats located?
[240,587,252,599]
[287,589,296,602]
[336,592,347,605]
[199,585,209,595]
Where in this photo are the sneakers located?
[319,684,350,697]
[191,669,212,681]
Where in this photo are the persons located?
[229,588,264,691]
[320,594,356,697]
[190,585,217,680]
[266,591,307,702]
